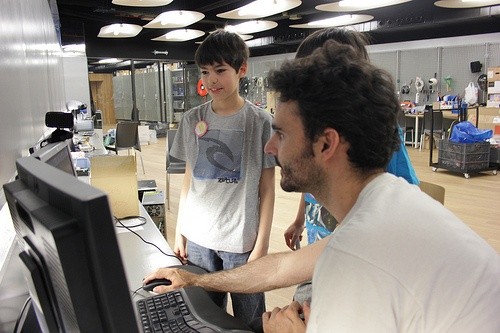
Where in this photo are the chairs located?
[165,129,186,210]
[104,121,145,175]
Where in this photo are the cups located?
[448,101,458,109]
[433,101,447,109]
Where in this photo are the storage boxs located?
[438,66,500,172]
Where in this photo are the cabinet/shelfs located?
[169,65,208,124]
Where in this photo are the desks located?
[0,129,182,333]
[403,114,424,149]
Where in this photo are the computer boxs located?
[142,191,167,241]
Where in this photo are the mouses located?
[143,279,172,291]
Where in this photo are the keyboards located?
[136,286,254,333]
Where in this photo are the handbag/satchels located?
[464,82,478,106]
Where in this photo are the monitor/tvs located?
[3,139,139,333]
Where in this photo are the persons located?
[285,27,420,320]
[169,30,277,324]
[142,38,500,333]
